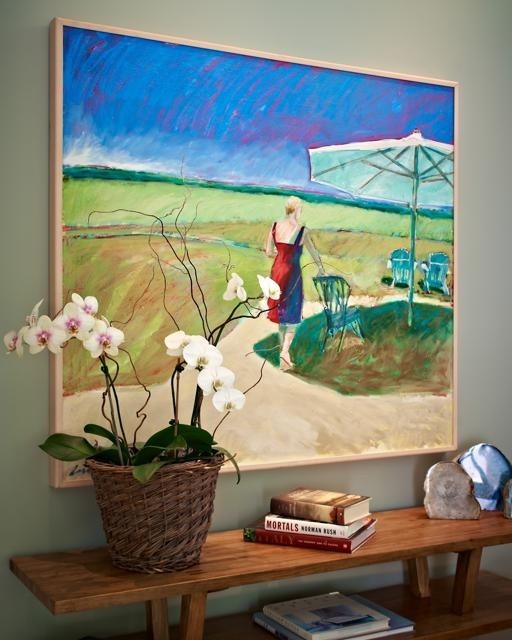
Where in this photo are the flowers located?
[3,138,286,484]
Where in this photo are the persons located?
[262,194,329,371]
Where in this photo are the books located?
[261,591,391,639]
[264,513,373,540]
[269,485,373,525]
[249,593,416,640]
[243,512,378,555]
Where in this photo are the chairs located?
[387,248,418,289]
[418,251,450,296]
[312,274,365,354]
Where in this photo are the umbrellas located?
[306,127,455,328]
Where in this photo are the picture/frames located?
[48,15,458,490]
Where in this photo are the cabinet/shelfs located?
[9,493,512,640]
